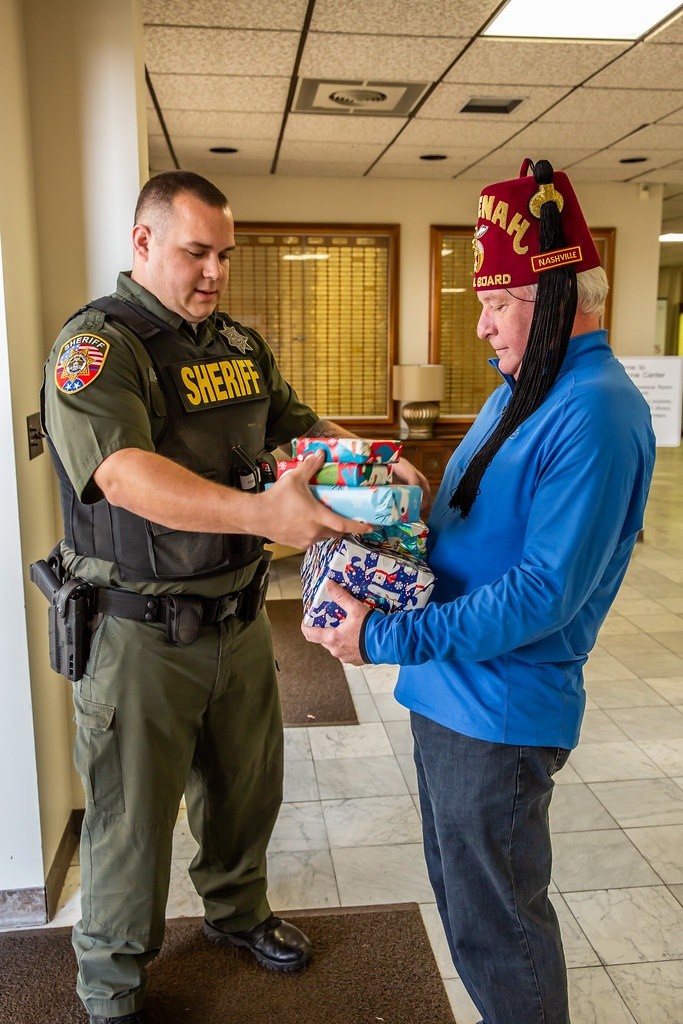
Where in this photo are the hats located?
[471,170,602,292]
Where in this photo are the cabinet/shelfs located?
[318,427,476,524]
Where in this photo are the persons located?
[296,155,661,1023]
[43,168,435,1024]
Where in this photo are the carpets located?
[0,900,458,1024]
[260,600,362,727]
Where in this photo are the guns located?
[29,560,88,682]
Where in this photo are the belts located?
[96,562,270,625]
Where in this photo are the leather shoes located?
[201,912,314,973]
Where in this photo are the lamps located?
[282,255,330,261]
[441,288,466,293]
[441,248,453,257]
